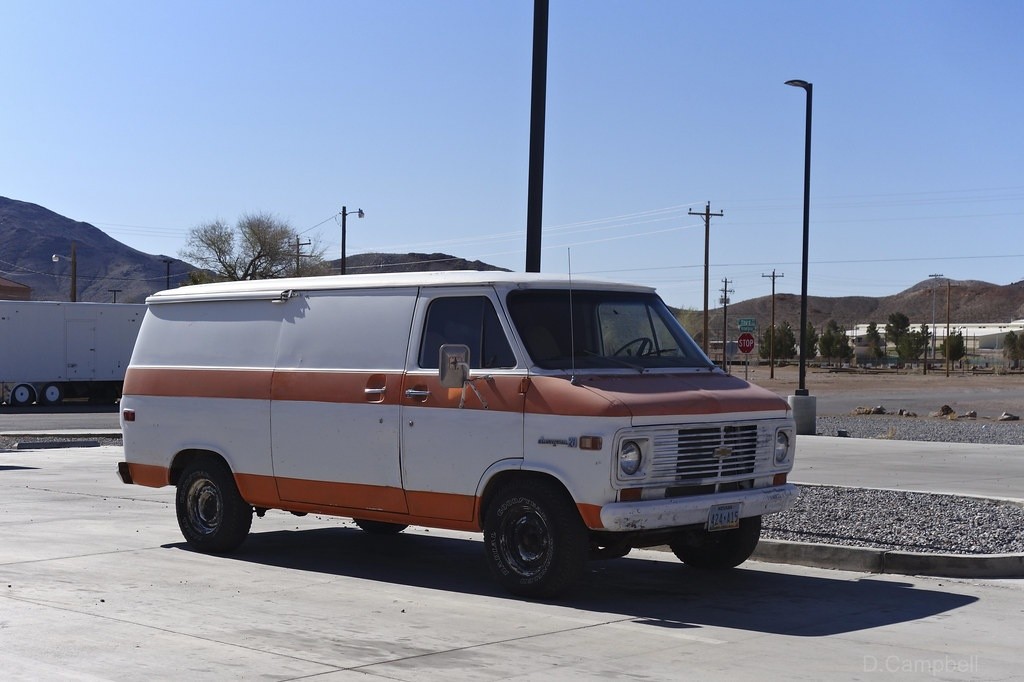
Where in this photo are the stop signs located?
[737,332,754,353]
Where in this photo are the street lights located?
[784,78,818,435]
[339,206,365,275]
[51,241,77,303]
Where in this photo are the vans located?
[118,271,802,604]
